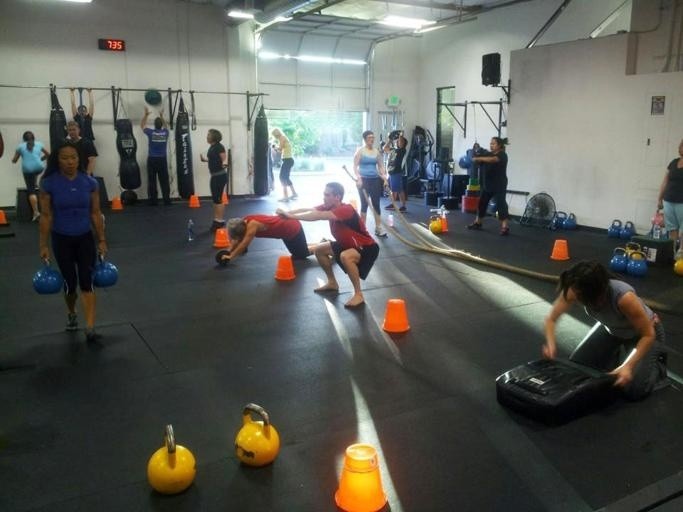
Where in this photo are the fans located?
[517,193,556,229]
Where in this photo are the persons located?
[542,259,667,402]
[658,137,683,258]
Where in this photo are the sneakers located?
[376,231,387,237]
[32,213,40,222]
[464,223,481,229]
[85,330,99,340]
[384,204,394,209]
[66,313,78,330]
[399,207,408,212]
[499,225,509,236]
[210,220,224,231]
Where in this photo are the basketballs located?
[145,91,162,103]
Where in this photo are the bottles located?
[388,212,399,228]
[649,222,667,241]
[186,219,194,241]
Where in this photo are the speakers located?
[481,53,501,86]
